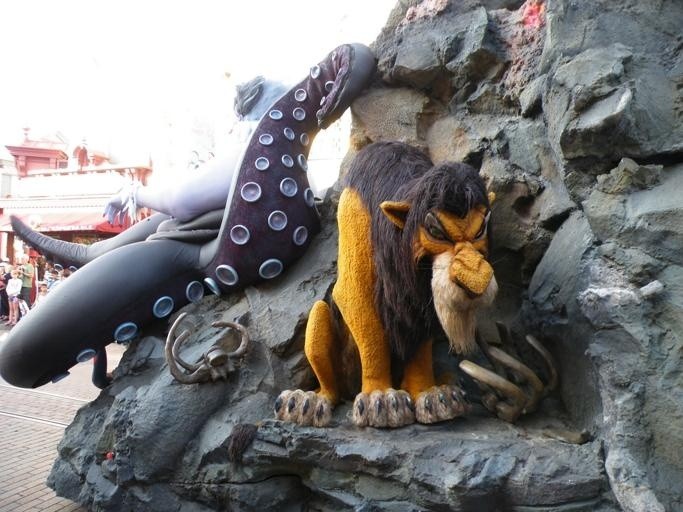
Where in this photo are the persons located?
[1,254,78,326]
